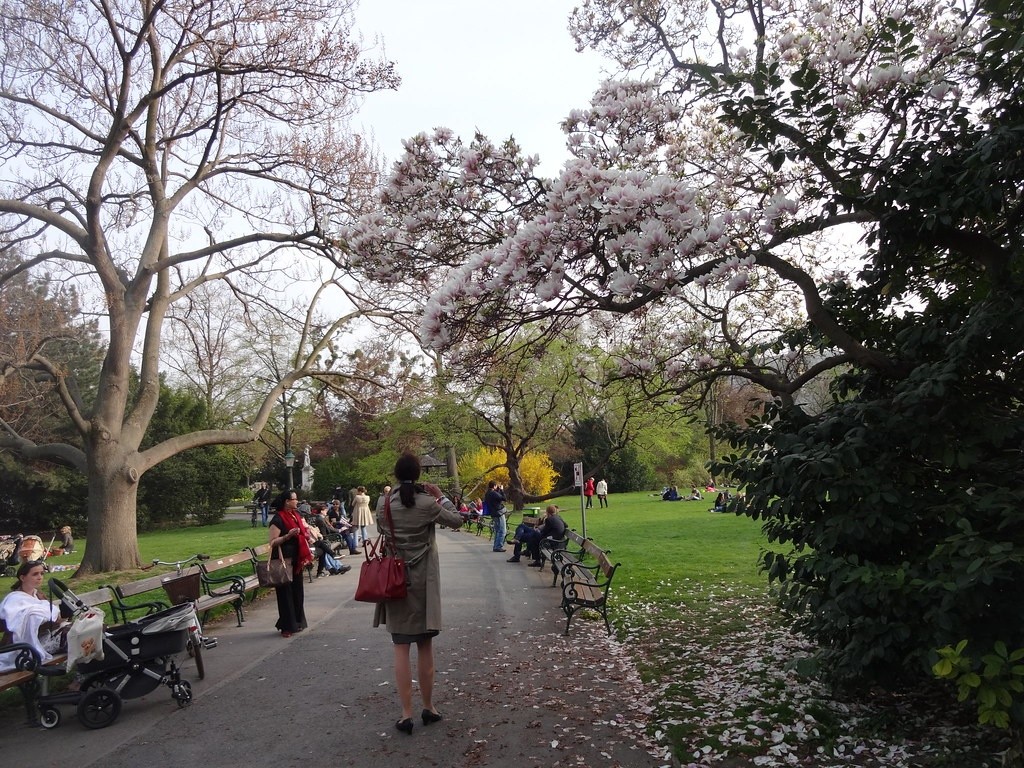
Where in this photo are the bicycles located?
[251,501,263,528]
[141,553,219,681]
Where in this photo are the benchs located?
[0,528,343,729]
[466,511,621,637]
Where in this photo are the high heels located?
[421,709,442,726]
[395,718,414,735]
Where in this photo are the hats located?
[590,477,595,481]
[63,526,71,530]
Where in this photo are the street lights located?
[285,449,295,490]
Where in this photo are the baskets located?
[160,569,201,607]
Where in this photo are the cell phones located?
[415,483,425,493]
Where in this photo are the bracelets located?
[436,495,446,502]
[283,535,287,540]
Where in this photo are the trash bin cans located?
[523,507,540,527]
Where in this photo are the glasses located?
[333,503,337,505]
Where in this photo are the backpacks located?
[584,483,588,490]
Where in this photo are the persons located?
[596,479,608,508]
[661,484,702,502]
[252,482,272,527]
[297,485,374,578]
[506,504,568,567]
[452,498,483,532]
[705,478,715,492]
[583,477,596,509]
[58,526,74,554]
[0,560,72,654]
[485,481,507,552]
[375,486,391,536]
[714,489,742,512]
[373,452,465,737]
[268,490,311,637]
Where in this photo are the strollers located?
[0,530,57,578]
[22,577,193,731]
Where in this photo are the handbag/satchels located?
[66,606,105,674]
[258,498,266,507]
[253,539,293,588]
[354,539,408,602]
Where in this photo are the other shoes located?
[591,505,593,509]
[493,548,506,552]
[340,565,351,574]
[281,630,291,637]
[519,549,531,557]
[263,522,267,527]
[507,555,520,562]
[318,572,329,578]
[329,568,339,575]
[333,554,345,559]
[587,506,590,508]
[350,550,362,554]
[528,561,542,567]
[507,538,520,545]
